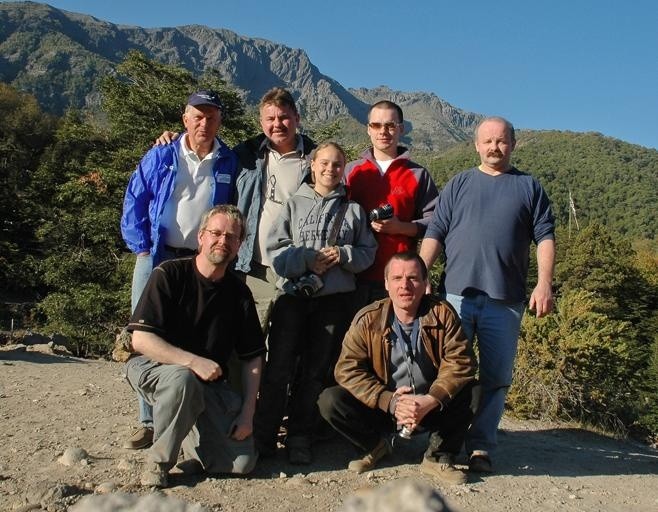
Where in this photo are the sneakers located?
[141,463,170,488]
[285,429,336,464]
[349,441,389,474]
[469,454,494,472]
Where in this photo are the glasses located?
[368,123,398,130]
[206,228,240,243]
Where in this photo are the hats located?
[188,89,221,110]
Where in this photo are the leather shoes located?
[123,425,154,450]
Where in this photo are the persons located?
[247,142,383,468]
[154,86,321,334]
[335,100,442,349]
[315,248,483,486]
[406,114,558,474]
[117,88,242,451]
[121,203,271,489]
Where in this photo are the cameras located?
[368,204,394,222]
[292,271,326,298]
